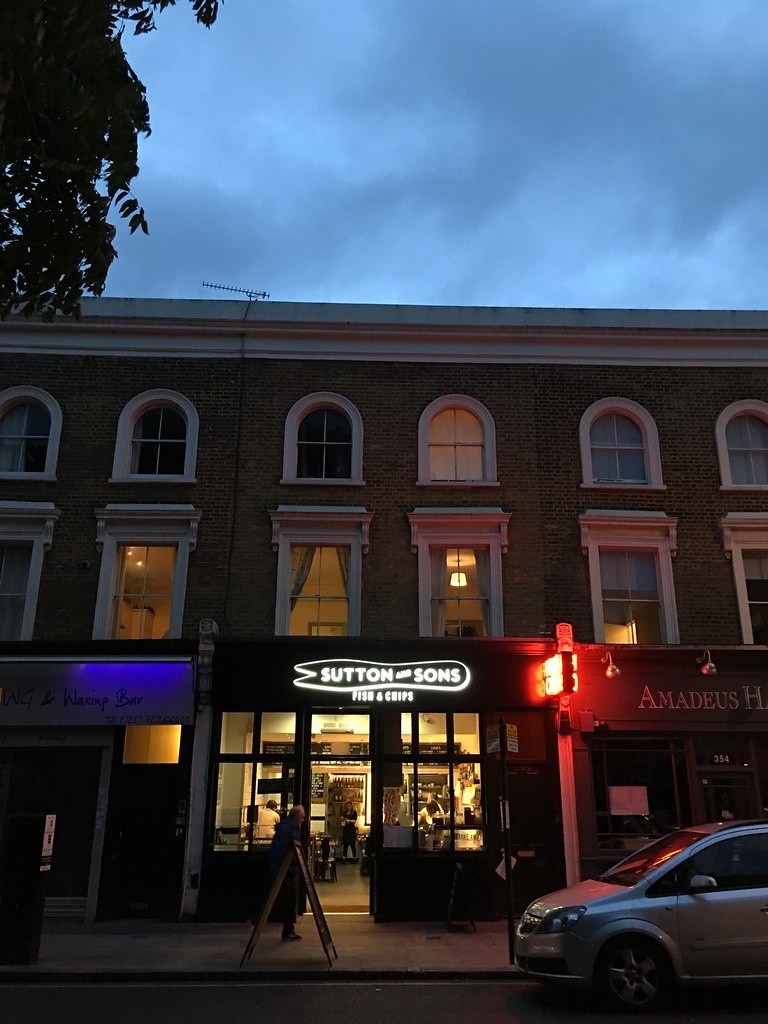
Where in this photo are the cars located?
[513,815,768,1014]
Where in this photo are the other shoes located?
[341,857,345,864]
[352,858,357,864]
[281,931,303,941]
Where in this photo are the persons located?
[257,799,280,837]
[420,793,445,845]
[268,805,307,939]
[340,802,357,863]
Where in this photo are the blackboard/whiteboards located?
[292,842,334,952]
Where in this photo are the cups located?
[425,835,433,851]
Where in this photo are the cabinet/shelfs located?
[324,772,366,827]
[408,773,449,816]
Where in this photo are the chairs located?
[314,837,337,883]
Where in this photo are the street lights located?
[538,651,585,887]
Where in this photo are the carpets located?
[308,904,370,913]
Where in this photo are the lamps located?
[601,651,622,678]
[694,648,718,675]
[450,547,468,588]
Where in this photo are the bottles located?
[333,777,364,802]
[217,831,223,844]
